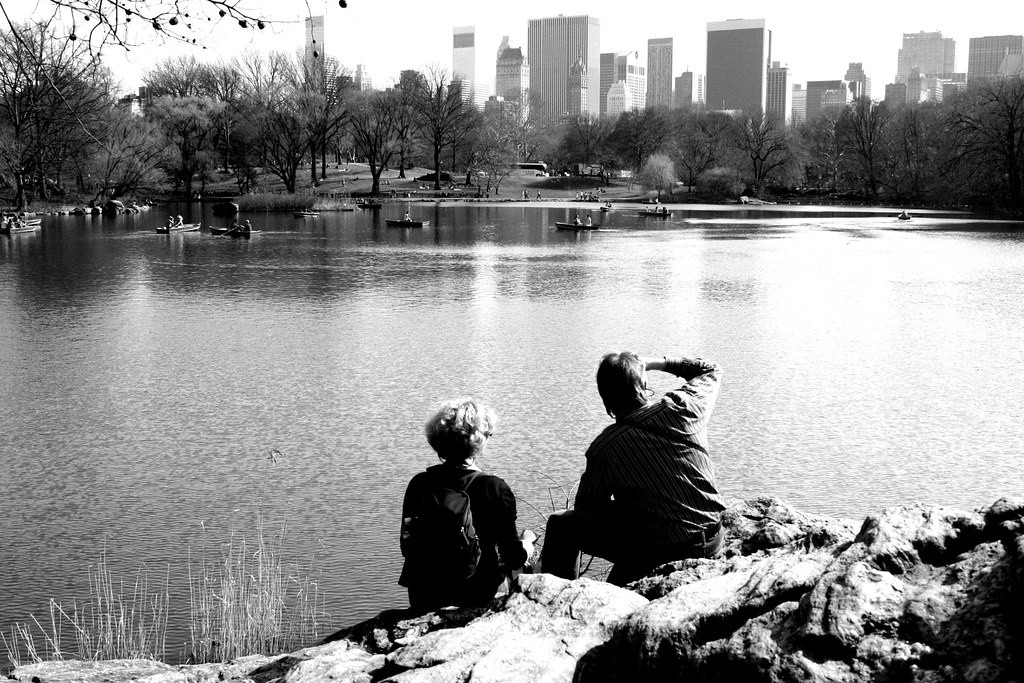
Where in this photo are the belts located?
[685,522,721,547]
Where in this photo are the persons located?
[404,211,412,221]
[541,168,565,177]
[243,219,251,231]
[521,189,524,199]
[574,187,668,226]
[434,191,451,197]
[410,177,418,182]
[145,197,152,203]
[301,205,311,213]
[363,199,375,205]
[903,210,907,217]
[133,199,136,204]
[230,219,239,230]
[165,216,174,228]
[476,189,486,198]
[464,171,472,188]
[525,189,529,199]
[383,180,390,185]
[403,191,410,198]
[537,190,541,200]
[449,184,454,189]
[540,349,726,584]
[0,211,27,228]
[326,163,331,167]
[175,215,183,227]
[397,395,541,616]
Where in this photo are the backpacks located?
[414,466,482,579]
